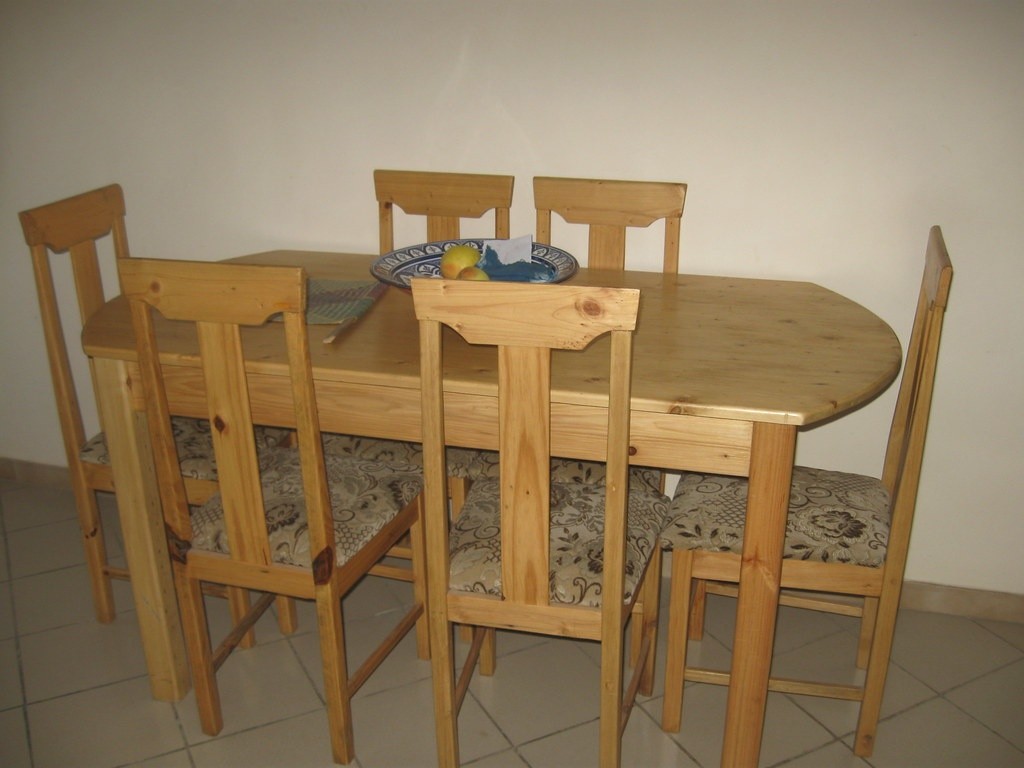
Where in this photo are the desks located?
[76,245,907,768]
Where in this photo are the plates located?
[369,238,580,298]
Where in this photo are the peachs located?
[439,245,490,281]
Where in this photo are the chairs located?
[405,274,669,768]
[119,243,423,765]
[463,176,690,489]
[17,179,288,649]
[306,165,516,509]
[653,219,959,758]
